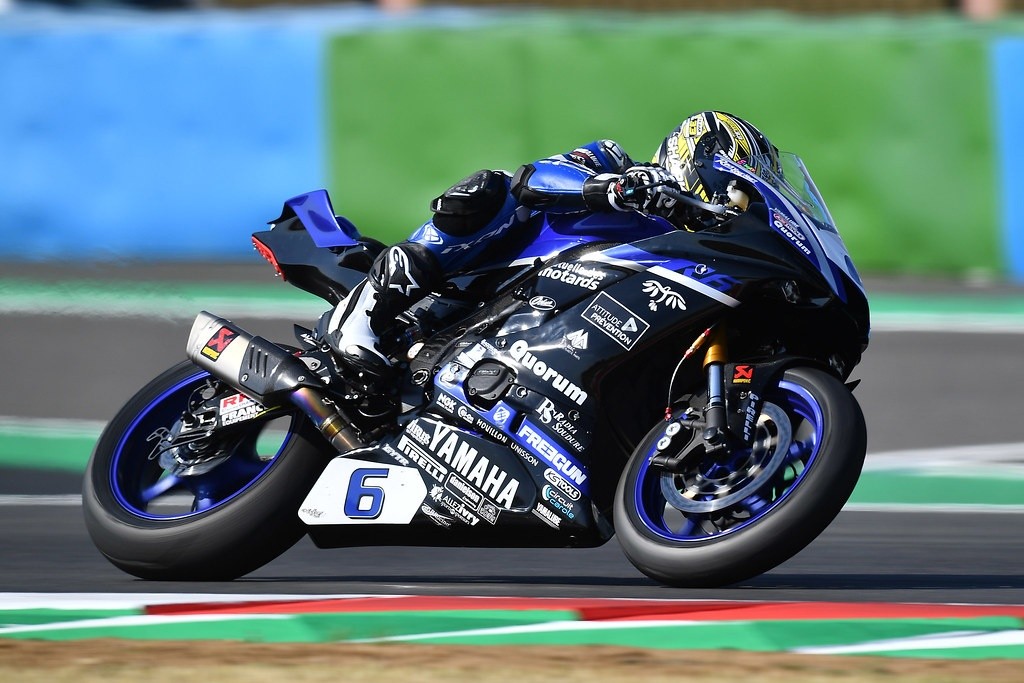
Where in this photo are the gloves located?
[583,162,680,219]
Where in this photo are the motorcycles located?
[78,144,875,589]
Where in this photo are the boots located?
[319,241,441,376]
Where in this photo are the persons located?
[309,111,786,375]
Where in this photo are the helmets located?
[649,109,783,229]
